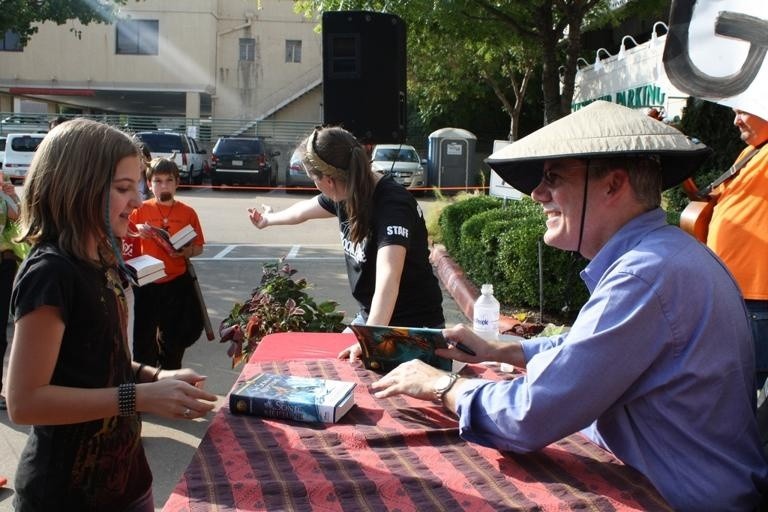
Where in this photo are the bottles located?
[472,284,500,366]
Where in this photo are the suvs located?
[130,128,207,191]
[207,137,282,191]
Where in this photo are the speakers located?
[323,13,407,142]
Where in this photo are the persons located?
[707,104,768,404]
[126,158,205,368]
[0,174,23,411]
[5,119,219,512]
[247,127,447,334]
[370,101,768,510]
[134,142,153,203]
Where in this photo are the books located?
[228,372,358,425]
[122,269,165,287]
[346,321,468,372]
[124,253,166,276]
[172,232,199,250]
[144,223,194,243]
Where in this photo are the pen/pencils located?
[421,325,476,359]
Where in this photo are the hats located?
[484,100,708,199]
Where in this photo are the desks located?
[162,332,677,511]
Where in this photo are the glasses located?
[543,167,614,184]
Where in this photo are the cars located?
[2,134,50,186]
[286,142,321,196]
[370,143,428,196]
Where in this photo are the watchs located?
[433,374,457,399]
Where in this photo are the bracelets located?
[136,364,164,381]
[115,382,140,420]
